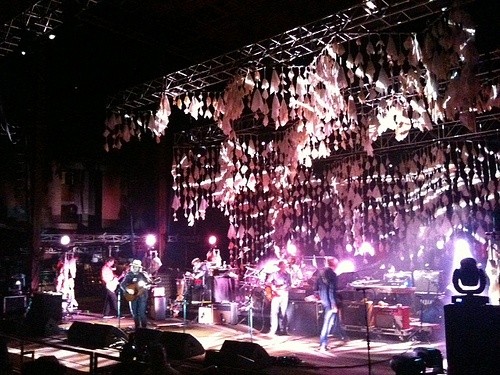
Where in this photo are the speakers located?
[125,327,162,354]
[65,321,94,343]
[29,294,63,335]
[217,339,274,369]
[452,257,488,294]
[158,331,208,360]
[86,324,129,348]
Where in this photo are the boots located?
[135,319,141,329]
[142,319,147,327]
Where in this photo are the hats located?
[128,259,143,268]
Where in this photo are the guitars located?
[124,277,161,302]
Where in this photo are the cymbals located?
[350,280,383,289]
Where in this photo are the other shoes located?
[104,316,115,319]
[117,316,126,319]
[341,336,352,341]
[279,328,288,336]
[320,346,331,351]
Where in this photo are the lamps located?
[451,257,487,295]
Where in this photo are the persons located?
[313,258,349,350]
[120,259,152,327]
[102,257,125,319]
[265,261,291,335]
[148,251,163,275]
[185,258,206,278]
[142,248,155,271]
